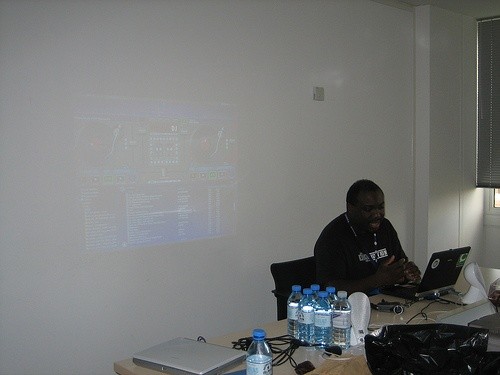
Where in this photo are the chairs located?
[270,256,315,321]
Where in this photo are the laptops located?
[385,246,471,298]
[132,337,250,375]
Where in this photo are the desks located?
[113,267,500,375]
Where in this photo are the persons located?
[314,180,421,298]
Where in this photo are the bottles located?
[332,290,352,350]
[246,329,273,375]
[287,285,304,339]
[326,287,338,303]
[298,288,314,347]
[311,284,320,301]
[313,291,333,350]
